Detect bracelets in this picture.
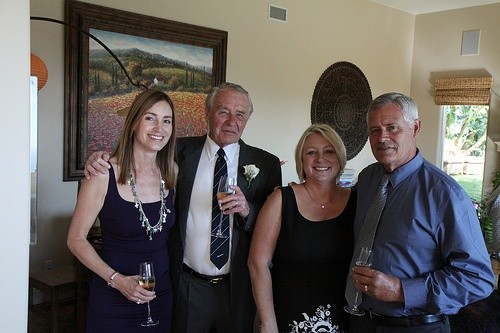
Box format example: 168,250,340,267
107,272,119,286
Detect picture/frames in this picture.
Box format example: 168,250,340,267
62,0,227,182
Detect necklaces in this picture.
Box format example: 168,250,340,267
127,174,171,241
321,206,325,209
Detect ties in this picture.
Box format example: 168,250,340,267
209,148,233,270
343,174,392,309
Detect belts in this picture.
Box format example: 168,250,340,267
364,315,444,329
183,264,232,286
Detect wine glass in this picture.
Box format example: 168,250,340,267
210,177,237,239
139,262,159,327
343,247,372,316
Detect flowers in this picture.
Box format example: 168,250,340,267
243,164,259,190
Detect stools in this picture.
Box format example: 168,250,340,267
27,267,72,333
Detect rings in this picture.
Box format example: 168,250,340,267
235,201,238,207
365,285,367,292
136,299,140,305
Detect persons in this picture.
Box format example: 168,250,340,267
83,66,282,333
67,91,180,333
247,123,357,333
288,92,495,333
